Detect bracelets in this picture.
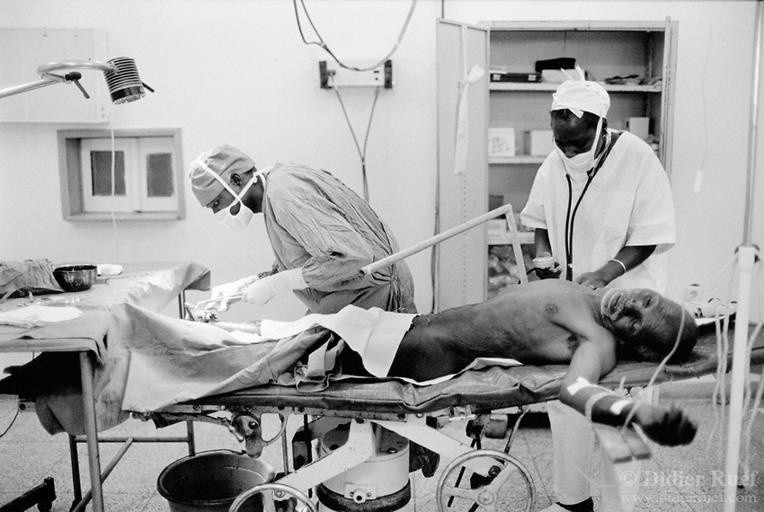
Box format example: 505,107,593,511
609,256,625,272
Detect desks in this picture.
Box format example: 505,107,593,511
0,265,211,511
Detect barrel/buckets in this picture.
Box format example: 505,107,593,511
155,448,275,512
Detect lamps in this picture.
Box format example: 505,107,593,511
0,57,154,106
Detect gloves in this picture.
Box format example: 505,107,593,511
203,272,262,315
240,267,308,308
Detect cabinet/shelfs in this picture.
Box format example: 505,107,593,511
434,18,677,319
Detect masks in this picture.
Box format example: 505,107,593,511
551,115,608,172
201,160,259,233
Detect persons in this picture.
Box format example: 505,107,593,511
328,280,700,448
189,144,415,316
517,81,677,510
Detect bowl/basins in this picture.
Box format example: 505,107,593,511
52,266,97,291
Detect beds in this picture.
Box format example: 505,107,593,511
134,317,755,512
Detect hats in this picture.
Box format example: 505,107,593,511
548,79,612,121
190,145,256,209
